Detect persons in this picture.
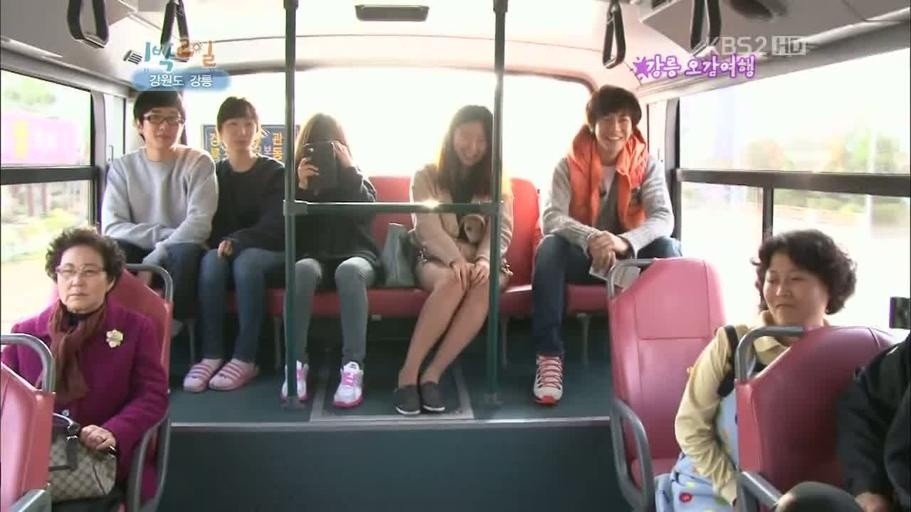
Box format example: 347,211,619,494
182,96,286,391
839,331,910,509
282,112,383,408
673,231,856,510
391,105,514,416
0,225,170,511
531,82,678,404
100,89,220,383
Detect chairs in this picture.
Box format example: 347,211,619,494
735,325,898,512
607,256,727,512
1,333,57,512
54,263,175,512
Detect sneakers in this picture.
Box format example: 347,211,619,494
420,379,446,413
180,358,223,394
531,354,565,406
391,375,419,416
332,367,365,409
208,356,261,391
281,360,307,403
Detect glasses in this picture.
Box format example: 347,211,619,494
141,112,184,127
52,264,106,277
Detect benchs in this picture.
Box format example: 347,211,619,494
152,177,623,368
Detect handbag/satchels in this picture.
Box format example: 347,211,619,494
45,410,117,501
380,221,413,291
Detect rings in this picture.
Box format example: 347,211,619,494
97,434,106,441
